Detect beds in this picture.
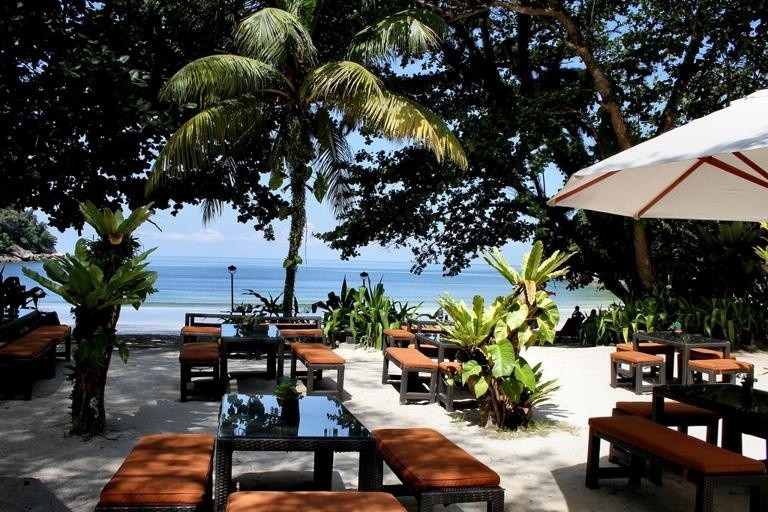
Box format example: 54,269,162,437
546,87,767,229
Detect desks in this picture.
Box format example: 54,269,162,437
359,271,369,288
227,264,238,311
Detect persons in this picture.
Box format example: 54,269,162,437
571,306,585,322
583,309,597,326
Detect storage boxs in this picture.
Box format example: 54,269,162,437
96,431,217,512
175,313,347,402
584,320,767,512
225,488,408,511
369,426,505,512
383,317,493,411
0,310,72,398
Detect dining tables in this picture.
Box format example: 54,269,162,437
272,376,304,414
